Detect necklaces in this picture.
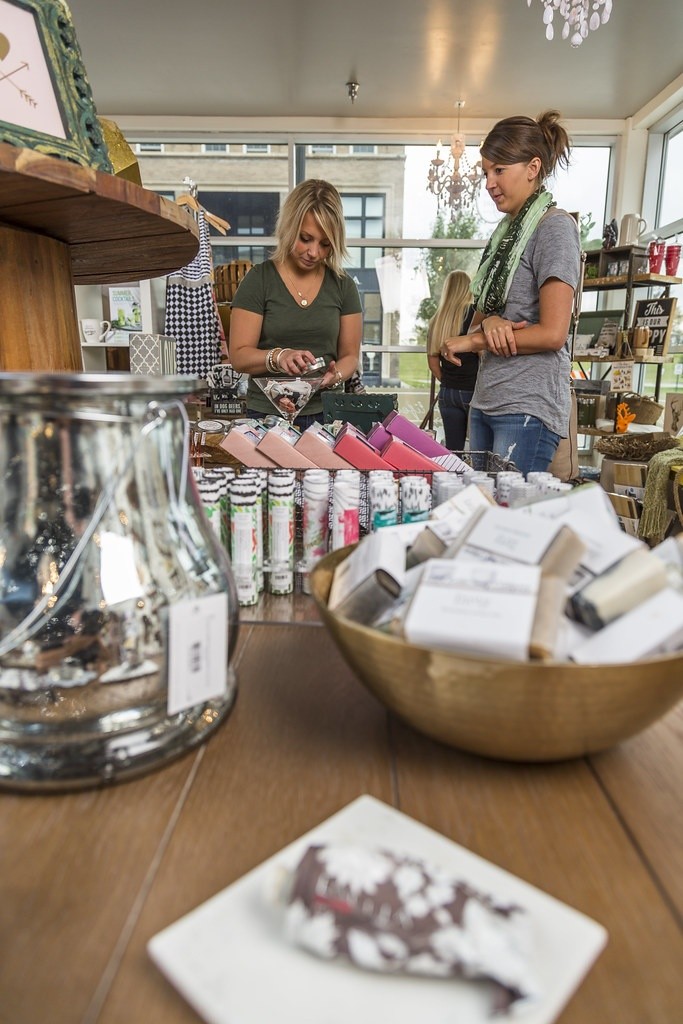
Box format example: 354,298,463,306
283,263,320,307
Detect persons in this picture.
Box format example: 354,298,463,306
438,110,581,488
426,270,478,458
229,179,363,432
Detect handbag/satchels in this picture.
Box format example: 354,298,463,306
607,392,664,425
545,388,580,483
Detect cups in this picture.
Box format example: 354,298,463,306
633,326,651,347
665,246,681,275
649,242,665,274
80,319,112,342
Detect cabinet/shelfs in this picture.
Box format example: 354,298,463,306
569,246,683,437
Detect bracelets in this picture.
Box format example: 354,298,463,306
266,348,291,374
480,322,484,333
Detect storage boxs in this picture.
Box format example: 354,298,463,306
219,409,477,468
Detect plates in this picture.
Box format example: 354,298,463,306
144,795,610,1024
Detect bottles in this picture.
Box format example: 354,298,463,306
0,370,239,792
619,214,647,245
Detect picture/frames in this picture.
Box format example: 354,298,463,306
0,1,113,175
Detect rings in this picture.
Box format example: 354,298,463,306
444,353,448,356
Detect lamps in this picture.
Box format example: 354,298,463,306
426,100,484,210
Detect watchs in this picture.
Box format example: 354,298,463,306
327,372,342,390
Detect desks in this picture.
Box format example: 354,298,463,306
0,593,682,1024
0,142,200,372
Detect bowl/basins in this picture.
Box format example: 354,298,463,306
307,543,682,763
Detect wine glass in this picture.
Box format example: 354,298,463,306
253,377,324,427
